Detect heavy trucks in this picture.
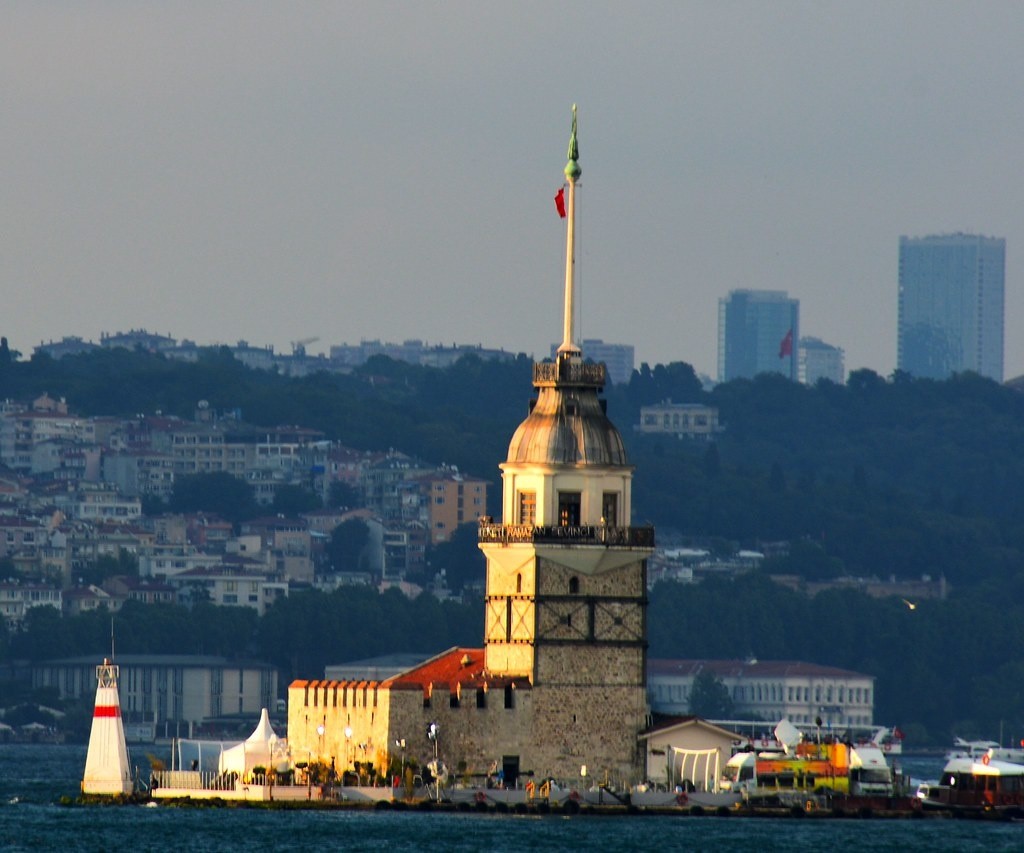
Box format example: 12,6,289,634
713,741,850,810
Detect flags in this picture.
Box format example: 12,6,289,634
554,188,568,219
779,330,792,358
895,729,907,741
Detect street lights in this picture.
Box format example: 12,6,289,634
429,721,440,803
344,726,354,771
317,725,326,762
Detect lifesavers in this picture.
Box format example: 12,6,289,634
675,791,689,805
569,791,580,801
911,797,922,809
476,791,487,801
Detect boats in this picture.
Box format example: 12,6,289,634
946,739,1024,763
914,756,1024,822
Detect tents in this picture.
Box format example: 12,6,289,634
218,709,287,774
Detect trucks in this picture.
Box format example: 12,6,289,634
848,745,894,799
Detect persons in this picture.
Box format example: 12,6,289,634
709,774,715,793
720,775,732,793
